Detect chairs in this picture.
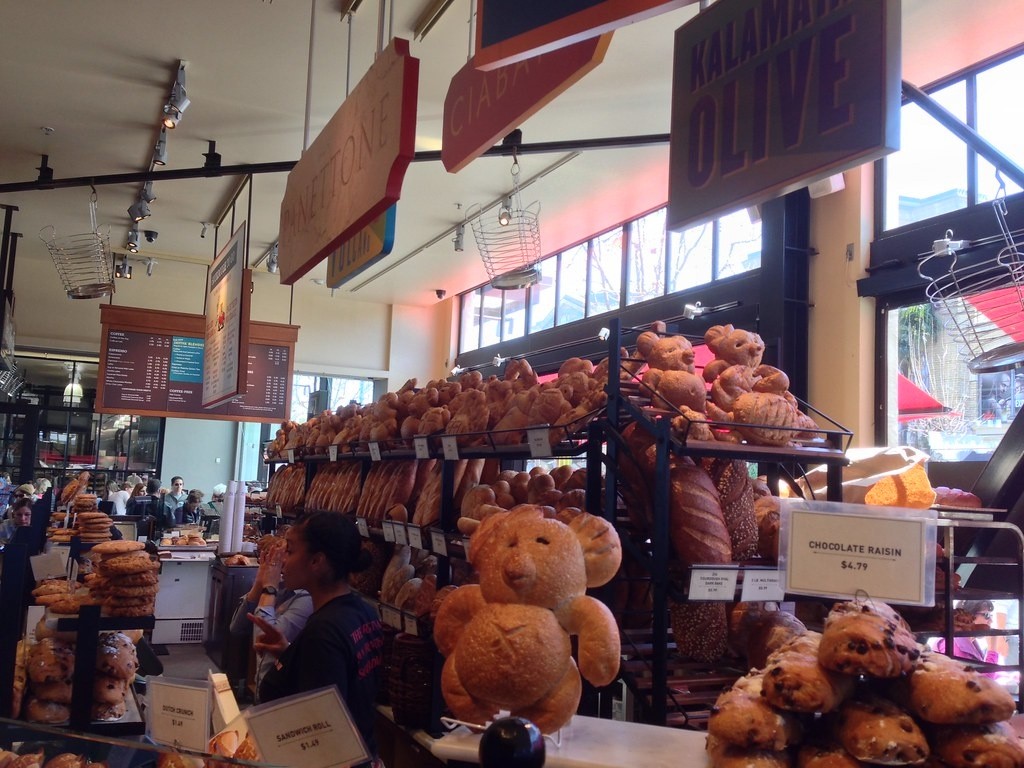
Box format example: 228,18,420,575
98,500,115,515
200,515,220,533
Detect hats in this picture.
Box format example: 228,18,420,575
186,493,201,503
213,483,227,496
142,475,148,480
147,479,162,491
14,484,34,496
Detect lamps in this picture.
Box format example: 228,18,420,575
497,194,514,226
266,241,280,274
452,226,465,252
63,368,83,408
114,83,190,278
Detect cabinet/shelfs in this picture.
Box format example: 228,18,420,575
254,319,854,728
4,605,164,744
930,504,1024,715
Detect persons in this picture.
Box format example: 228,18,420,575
1014,372,1024,400
981,373,1011,423
101,472,208,526
0,471,53,549
932,598,1002,682
209,481,227,505
228,510,390,768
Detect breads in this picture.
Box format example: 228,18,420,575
160,534,207,547
0,610,210,768
218,323,819,734
703,487,1024,768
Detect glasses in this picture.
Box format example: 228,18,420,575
14,494,26,498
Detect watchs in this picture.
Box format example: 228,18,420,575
259,585,277,597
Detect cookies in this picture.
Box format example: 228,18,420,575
30,480,159,619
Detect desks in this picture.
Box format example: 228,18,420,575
431,714,709,768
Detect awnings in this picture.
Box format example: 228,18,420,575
898,370,955,424
